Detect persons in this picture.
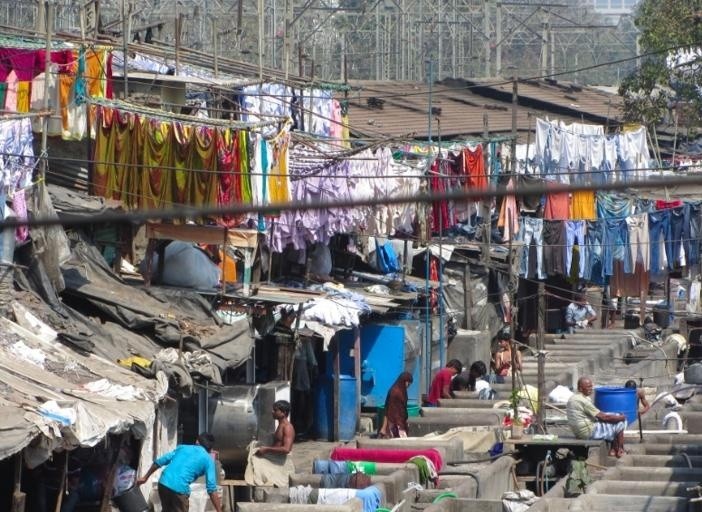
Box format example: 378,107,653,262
496,332,523,377
256,401,295,457
135,432,221,512
427,358,464,408
666,328,688,372
452,361,487,391
643,316,661,341
566,376,624,456
624,381,649,417
380,372,413,438
476,375,490,399
566,292,597,330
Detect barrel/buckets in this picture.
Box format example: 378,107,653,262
376,404,422,434
594,386,638,426
314,372,357,442
